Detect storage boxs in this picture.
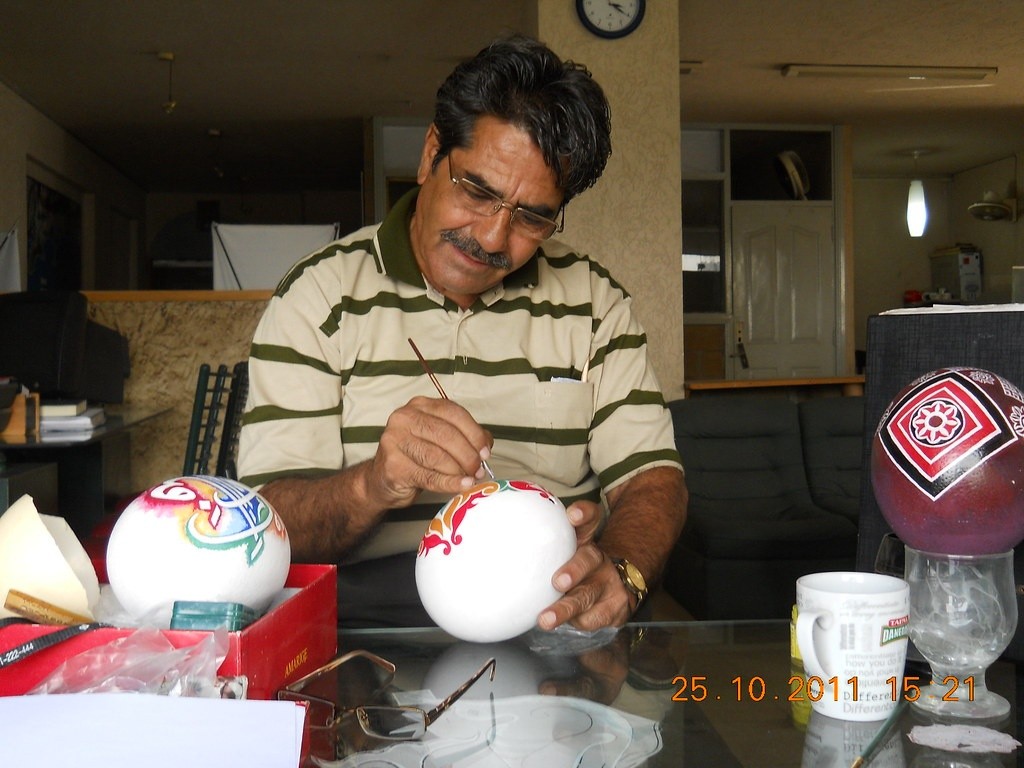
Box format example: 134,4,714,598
0,555,339,768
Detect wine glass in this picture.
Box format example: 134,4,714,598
903,544,1020,719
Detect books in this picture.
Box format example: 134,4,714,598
38,400,106,443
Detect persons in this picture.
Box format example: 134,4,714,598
236,35,695,635
540,628,684,768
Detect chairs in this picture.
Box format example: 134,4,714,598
659,387,859,621
183,360,249,487
799,391,866,519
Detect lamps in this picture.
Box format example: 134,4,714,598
782,64,998,83
894,146,940,238
158,51,177,114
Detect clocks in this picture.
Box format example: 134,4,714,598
576,0,646,40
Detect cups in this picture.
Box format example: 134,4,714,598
795,570,911,721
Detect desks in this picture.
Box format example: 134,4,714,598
0,402,177,540
310,624,1018,768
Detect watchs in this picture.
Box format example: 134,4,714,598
630,627,647,658
610,557,649,614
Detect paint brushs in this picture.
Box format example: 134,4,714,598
408,337,498,480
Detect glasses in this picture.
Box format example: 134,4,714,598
276,650,498,741
447,150,565,243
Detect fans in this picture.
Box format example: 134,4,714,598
967,186,1018,224
